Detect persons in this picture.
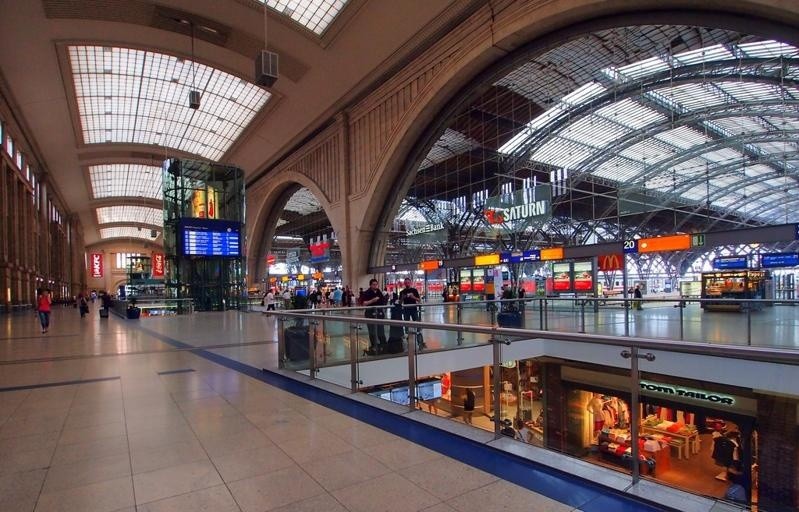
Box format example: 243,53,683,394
514,420,535,443
256,278,427,349
602,397,627,427
427,401,438,414
634,285,644,310
37,288,114,334
441,373,449,394
193,191,200,215
442,278,526,311
461,384,477,424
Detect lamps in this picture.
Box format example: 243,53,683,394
188,24,201,109
253,0,281,90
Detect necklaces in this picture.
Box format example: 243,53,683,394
501,419,516,437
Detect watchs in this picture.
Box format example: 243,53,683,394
586,394,604,434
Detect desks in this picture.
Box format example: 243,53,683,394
641,421,700,460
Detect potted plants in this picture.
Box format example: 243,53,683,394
497,288,521,328
126,297,141,319
283,292,316,361
